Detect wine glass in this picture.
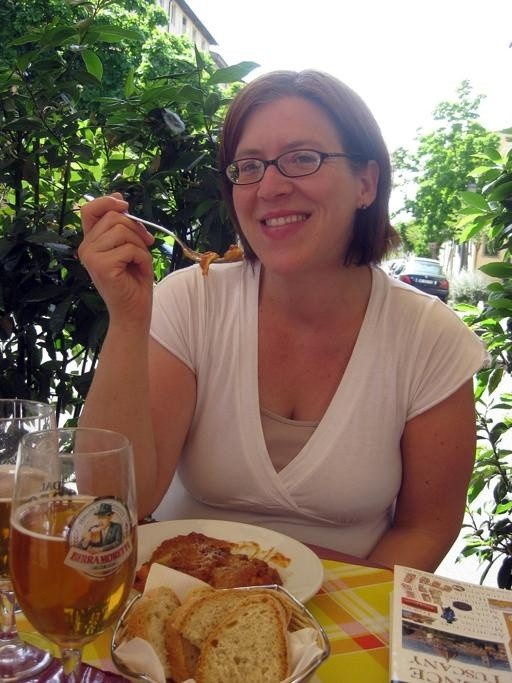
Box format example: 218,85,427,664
9,427,137,683
0,397,61,683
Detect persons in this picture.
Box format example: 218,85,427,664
74,70,488,579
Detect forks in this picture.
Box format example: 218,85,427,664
84,195,244,265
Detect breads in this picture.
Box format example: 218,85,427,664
115,583,292,683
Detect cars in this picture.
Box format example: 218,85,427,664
374,252,449,307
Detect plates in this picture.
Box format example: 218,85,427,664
132,519,323,606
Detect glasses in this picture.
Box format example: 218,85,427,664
225,148,367,185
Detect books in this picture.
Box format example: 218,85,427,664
386,560,512,683
